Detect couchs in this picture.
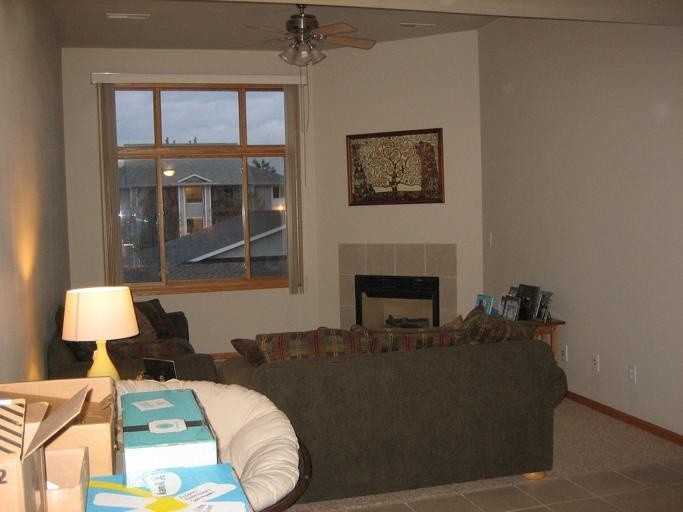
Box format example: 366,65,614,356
215,339,573,505
46,312,218,382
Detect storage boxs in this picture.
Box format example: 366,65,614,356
0,377,256,512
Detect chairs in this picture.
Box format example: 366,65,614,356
116,378,313,512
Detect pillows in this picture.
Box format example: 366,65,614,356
55,298,196,365
230,303,527,364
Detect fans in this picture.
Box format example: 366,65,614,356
233,3,377,50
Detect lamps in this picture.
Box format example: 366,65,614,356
62,286,141,379
278,40,326,66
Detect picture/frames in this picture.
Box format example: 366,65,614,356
345,127,445,206
476,284,554,322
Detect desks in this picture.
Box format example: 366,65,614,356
516,317,565,366
